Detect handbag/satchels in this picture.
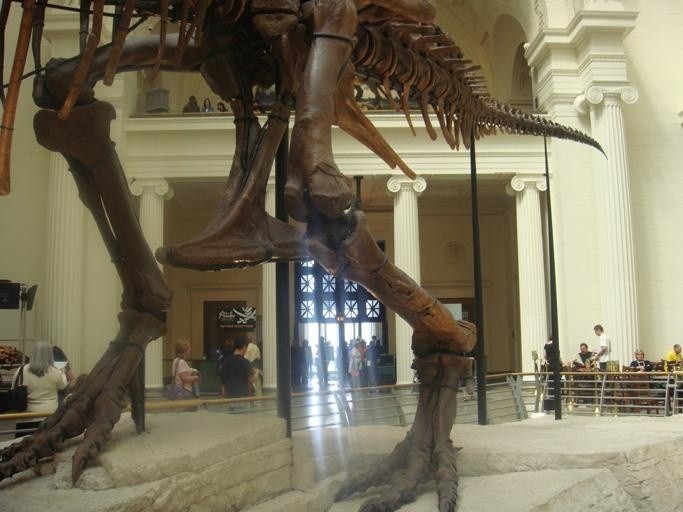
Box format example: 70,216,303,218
1,365,27,413
166,385,198,412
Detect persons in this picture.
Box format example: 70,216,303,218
290,335,383,387
666,343,683,365
216,336,263,398
12,341,68,439
591,325,610,370
182,95,228,113
572,343,596,368
172,340,200,399
52,345,75,403
630,349,652,371
543,335,563,395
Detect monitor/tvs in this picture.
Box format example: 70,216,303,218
0,283,20,309
26,285,37,310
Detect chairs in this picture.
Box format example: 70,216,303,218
539,358,683,415
